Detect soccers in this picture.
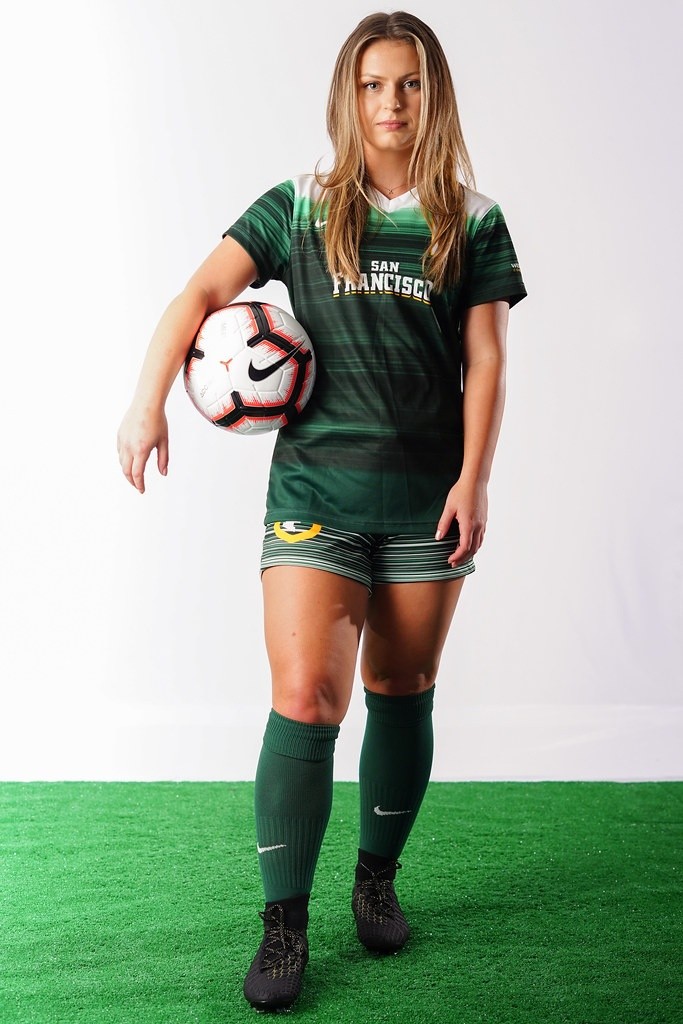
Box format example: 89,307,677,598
183,301,315,436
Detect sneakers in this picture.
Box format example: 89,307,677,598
347,847,410,951
243,893,312,1010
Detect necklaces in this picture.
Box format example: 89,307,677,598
373,182,407,194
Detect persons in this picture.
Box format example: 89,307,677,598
116,11,527,1010
